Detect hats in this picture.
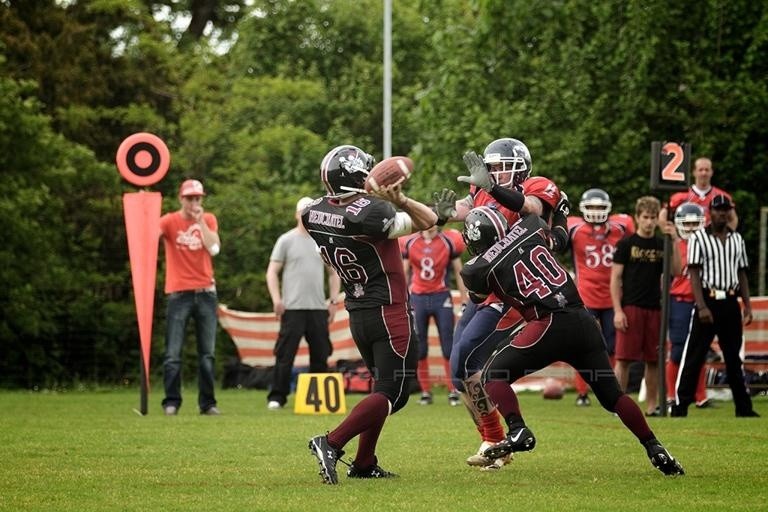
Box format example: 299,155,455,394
297,197,315,212
181,178,204,199
709,195,734,211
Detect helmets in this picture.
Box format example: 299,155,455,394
460,206,512,255
320,144,375,203
675,203,705,241
578,189,613,225
484,137,532,191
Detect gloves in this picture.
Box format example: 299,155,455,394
458,152,494,194
434,188,458,220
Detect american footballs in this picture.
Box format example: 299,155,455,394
365,156,414,195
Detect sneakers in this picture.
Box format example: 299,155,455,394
484,427,536,459
417,394,461,406
647,445,684,476
646,400,761,420
479,453,514,470
347,461,401,478
467,440,508,466
575,394,591,407
309,434,345,486
163,401,287,418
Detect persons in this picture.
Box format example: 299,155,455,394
266,138,684,488
658,157,761,419
159,179,221,416
